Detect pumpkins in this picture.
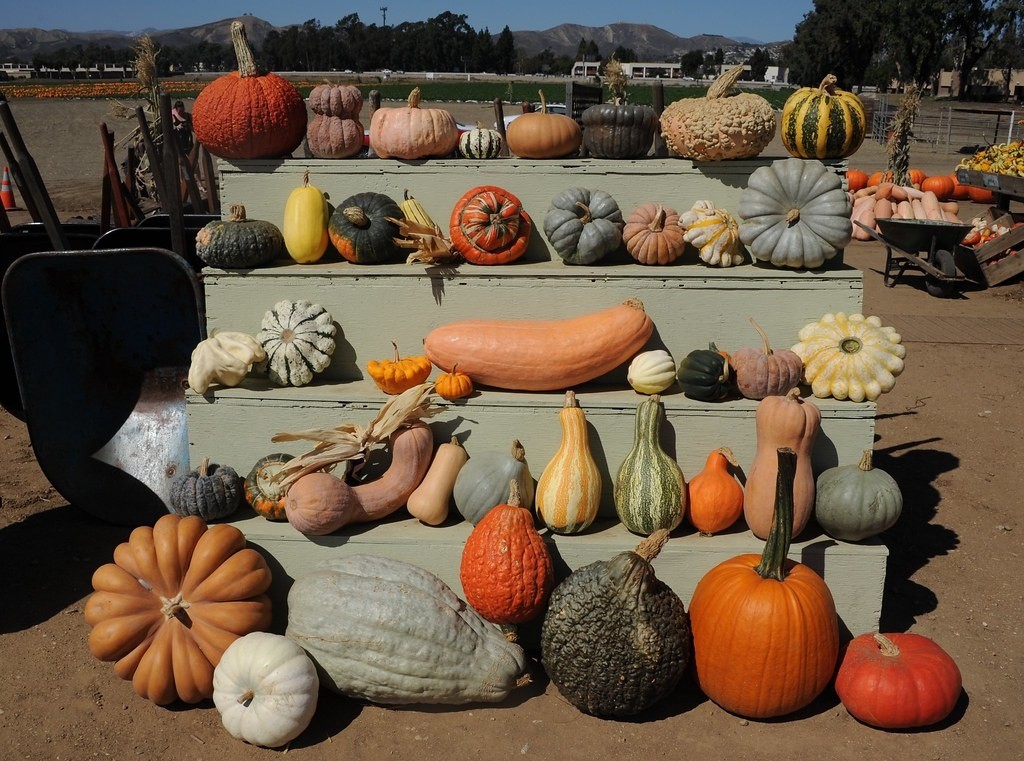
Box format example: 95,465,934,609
85,22,1023,744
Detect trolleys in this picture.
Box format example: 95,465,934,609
853,217,977,298
956,169,1024,213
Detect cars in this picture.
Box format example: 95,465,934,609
494,103,567,133
683,75,695,81
381,68,393,74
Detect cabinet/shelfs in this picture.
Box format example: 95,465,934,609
182,152,891,639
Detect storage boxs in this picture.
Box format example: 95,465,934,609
959,224,1024,287
964,205,1014,233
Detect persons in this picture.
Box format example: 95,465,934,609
171,100,209,193
